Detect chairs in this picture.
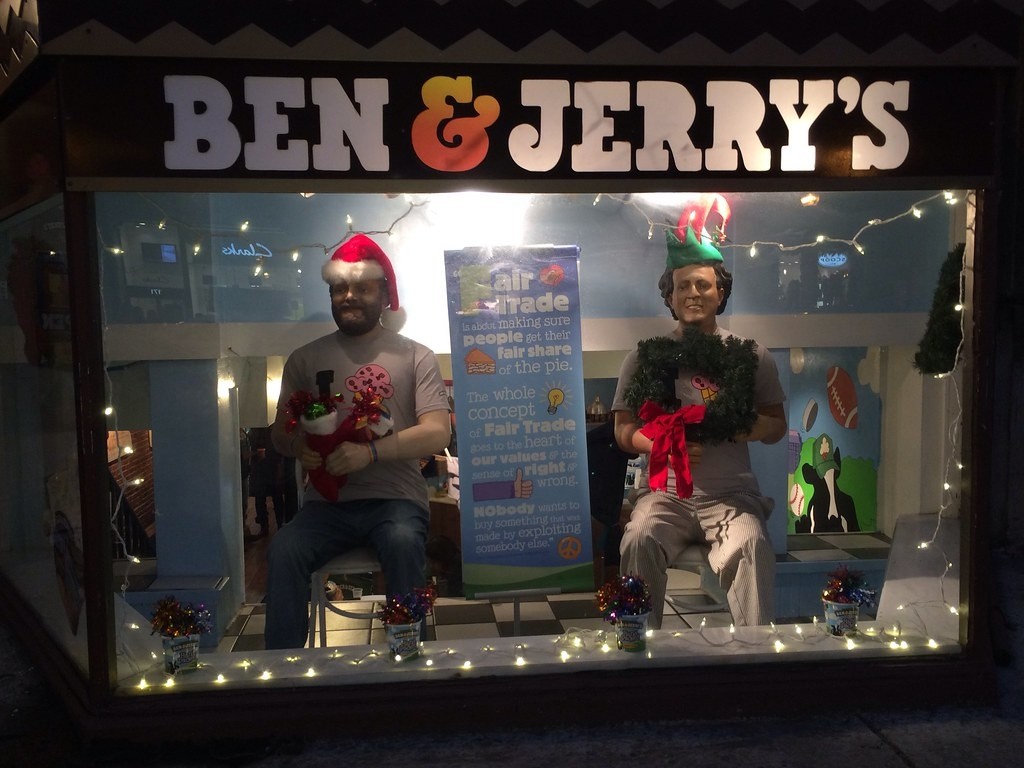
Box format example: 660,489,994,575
290,456,430,650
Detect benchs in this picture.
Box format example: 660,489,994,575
111,575,232,649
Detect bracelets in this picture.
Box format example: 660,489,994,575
366,441,381,464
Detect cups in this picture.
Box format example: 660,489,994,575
428,456,449,497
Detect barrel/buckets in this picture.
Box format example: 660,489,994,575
160,633,200,676
821,597,861,640
614,612,651,654
382,619,423,661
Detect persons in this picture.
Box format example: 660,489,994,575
611,217,789,627
262,235,453,651
248,428,284,538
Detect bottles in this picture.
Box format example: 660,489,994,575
255,447,266,463
